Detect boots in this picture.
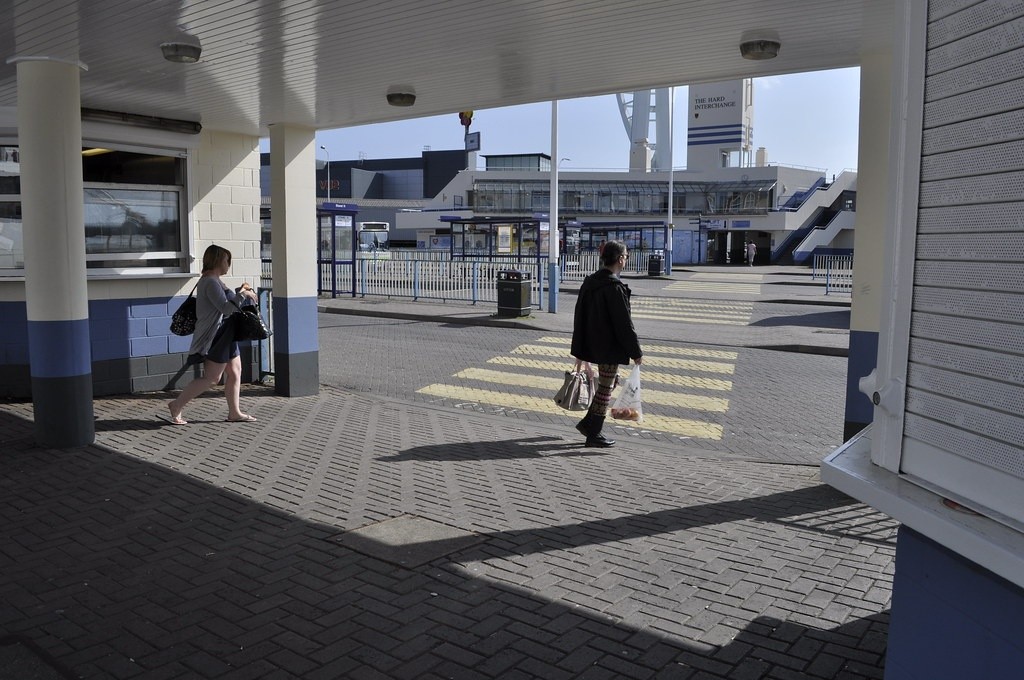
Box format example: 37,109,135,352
576,409,616,447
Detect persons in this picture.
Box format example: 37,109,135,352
570,239,644,448
748,240,756,267
156,245,259,424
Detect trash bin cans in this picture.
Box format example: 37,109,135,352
648,254,665,276
496,271,533,318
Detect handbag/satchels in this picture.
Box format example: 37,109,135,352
229,298,273,343
170,296,197,336
610,363,643,421
553,356,600,411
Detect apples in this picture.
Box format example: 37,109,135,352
611,407,639,420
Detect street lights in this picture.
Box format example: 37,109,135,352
320,145,331,203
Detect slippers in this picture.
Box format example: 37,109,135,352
153,414,187,426
224,414,257,422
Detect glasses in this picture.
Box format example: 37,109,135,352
622,253,629,259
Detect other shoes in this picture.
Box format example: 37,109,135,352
749,264,753,267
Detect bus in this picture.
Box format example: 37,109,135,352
262,222,391,263
